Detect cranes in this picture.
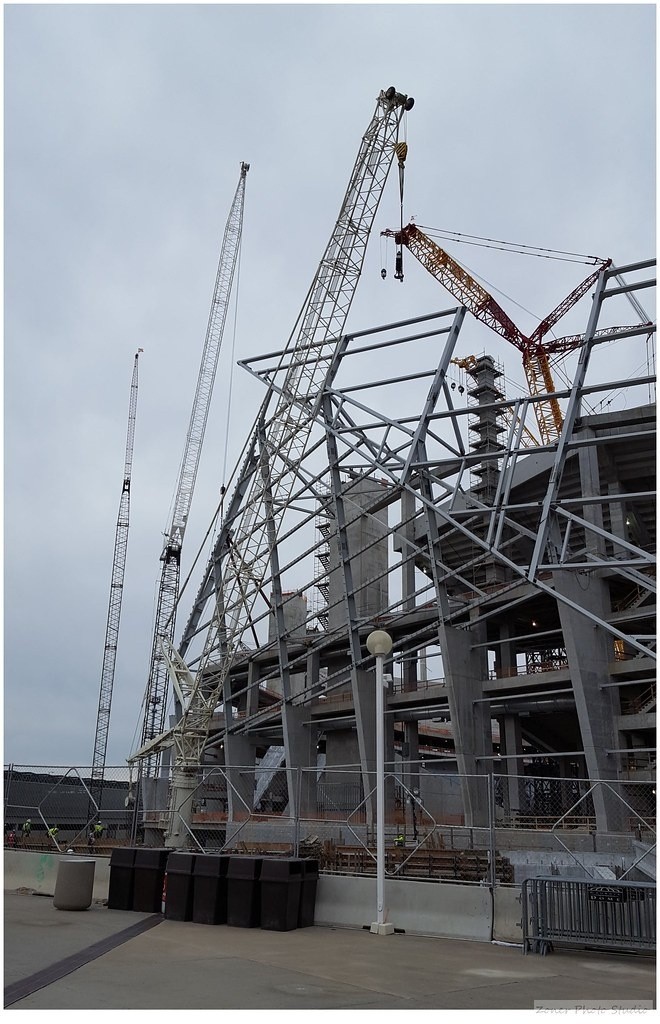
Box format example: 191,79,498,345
125,82,412,851
379,217,613,450
83,347,148,835
138,160,253,785
447,347,540,450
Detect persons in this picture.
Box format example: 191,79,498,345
45,827,60,839
94,820,104,839
22,818,32,839
8,829,18,849
87,832,96,854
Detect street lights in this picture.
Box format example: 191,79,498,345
358,629,396,936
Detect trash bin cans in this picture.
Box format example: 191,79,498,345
107,846,138,911
132,847,167,913
164,850,321,931
